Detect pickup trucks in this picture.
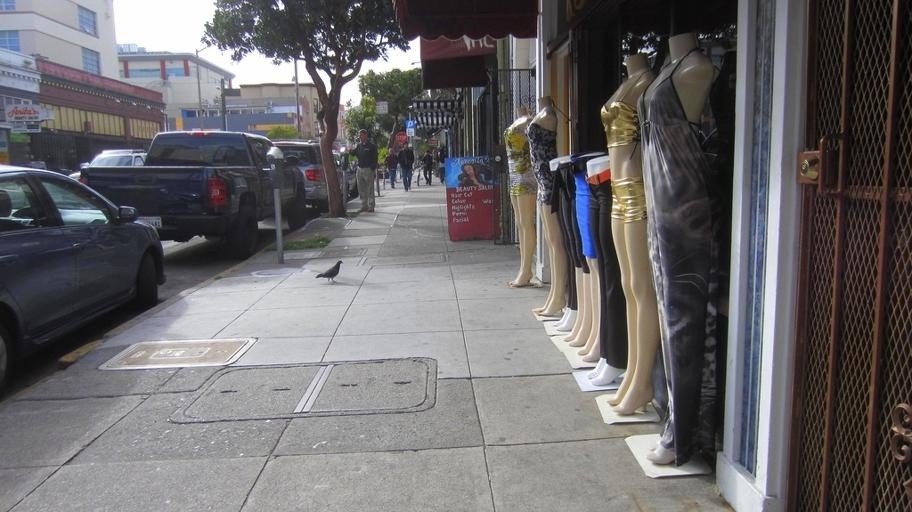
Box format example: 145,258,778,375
80,129,305,260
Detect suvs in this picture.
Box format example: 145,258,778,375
270,139,349,206
69,150,148,180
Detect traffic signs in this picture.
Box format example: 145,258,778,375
406,120,414,128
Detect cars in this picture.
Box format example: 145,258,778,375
0,164,164,395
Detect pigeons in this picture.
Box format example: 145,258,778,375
315,260,343,283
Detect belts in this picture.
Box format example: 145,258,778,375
585,169,611,186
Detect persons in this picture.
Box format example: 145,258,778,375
504,108,538,287
438,145,447,184
526,96,568,316
346,129,378,212
433,149,438,176
569,153,607,363
422,150,433,186
384,148,398,188
398,141,414,191
549,156,578,331
560,153,592,347
587,156,628,387
461,164,491,187
601,53,660,415
637,34,714,468
